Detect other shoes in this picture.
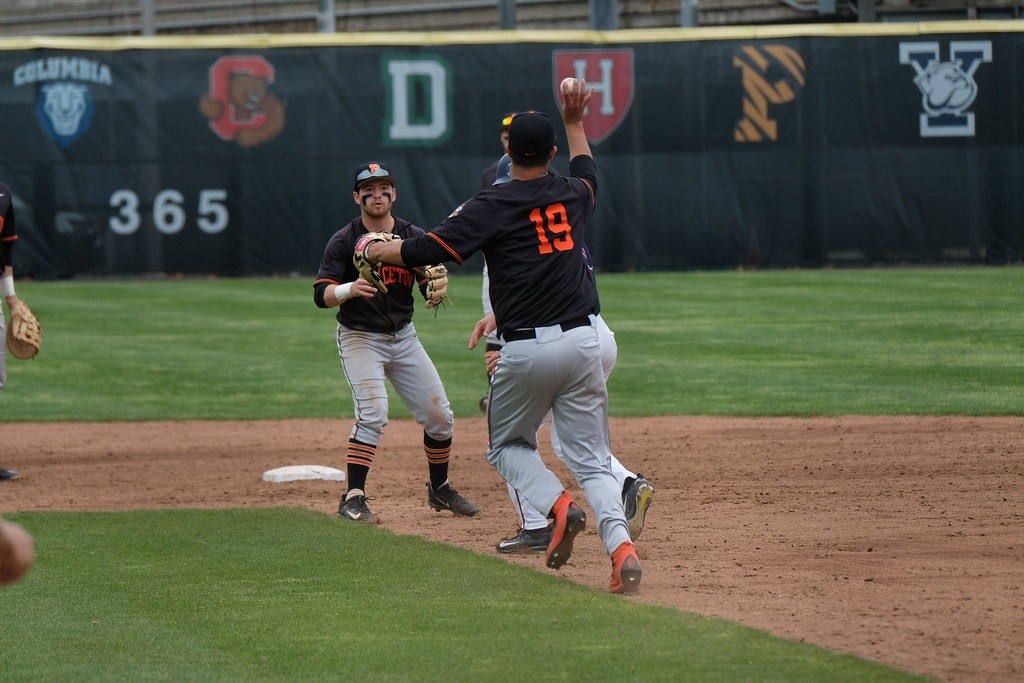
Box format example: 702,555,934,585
0,467,22,480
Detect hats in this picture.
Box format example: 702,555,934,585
507,110,557,158
500,112,519,133
353,162,394,192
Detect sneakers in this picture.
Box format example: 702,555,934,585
608,541,643,594
496,520,551,553
544,490,586,570
425,478,482,516
337,494,380,525
621,473,655,542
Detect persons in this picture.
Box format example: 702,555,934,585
0,181,43,480
0,516,34,587
353,77,657,594
312,161,480,522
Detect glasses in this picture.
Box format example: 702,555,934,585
501,113,518,127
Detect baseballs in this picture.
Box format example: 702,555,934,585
560,77,580,96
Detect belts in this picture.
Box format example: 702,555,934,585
503,319,582,343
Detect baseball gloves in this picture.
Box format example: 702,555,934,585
413,262,455,320
6,299,44,363
351,221,414,294
